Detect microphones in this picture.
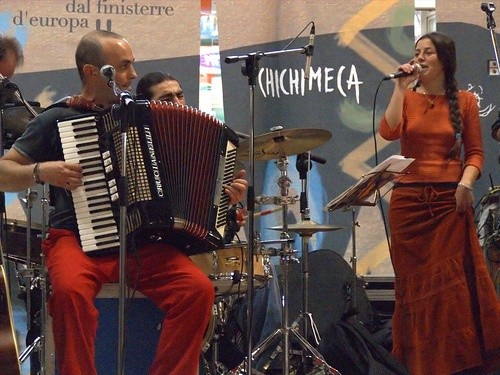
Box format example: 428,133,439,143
384,64,422,80
304,26,315,80
100,65,116,78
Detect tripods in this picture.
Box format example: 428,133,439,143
221,149,344,375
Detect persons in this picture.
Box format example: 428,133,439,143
378,32,500,375
136,71,283,375
0,30,248,375
0,36,23,80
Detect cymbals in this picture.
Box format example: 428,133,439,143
236,128,332,160
265,217,345,237
0,107,48,150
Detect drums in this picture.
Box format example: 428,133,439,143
0,218,48,265
188,239,273,296
0,264,21,375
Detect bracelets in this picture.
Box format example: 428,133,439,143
458,183,473,190
33,162,44,185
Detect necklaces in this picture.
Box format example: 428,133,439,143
421,88,444,108
